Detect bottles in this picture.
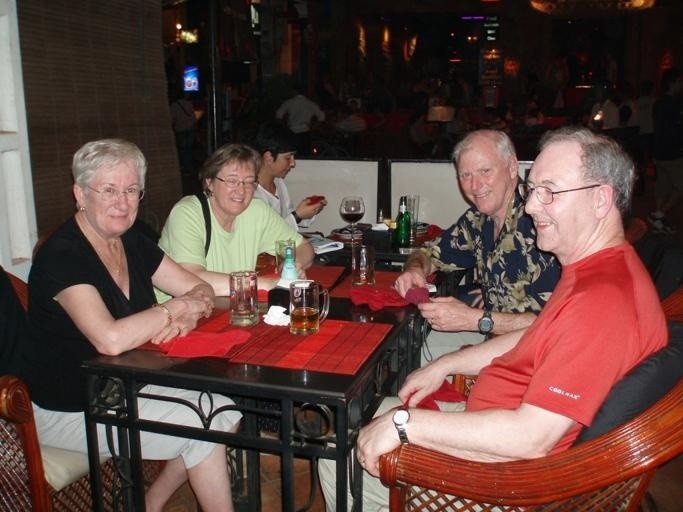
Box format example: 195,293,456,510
395,196,410,248
281,247,298,281
378,208,384,226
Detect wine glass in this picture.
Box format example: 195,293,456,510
338,195,364,247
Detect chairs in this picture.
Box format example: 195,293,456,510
0,267,166,512
379,283,682,512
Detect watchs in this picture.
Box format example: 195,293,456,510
392,406,415,444
479,310,493,336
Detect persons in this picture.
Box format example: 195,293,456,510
319,126,669,512
7,138,242,512
154,143,315,308
395,130,561,364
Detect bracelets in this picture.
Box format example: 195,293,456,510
151,302,172,324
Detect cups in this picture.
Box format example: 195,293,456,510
288,281,330,336
350,240,376,287
268,288,289,315
405,195,418,243
275,239,296,278
228,271,259,327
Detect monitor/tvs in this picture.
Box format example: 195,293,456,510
182,62,201,92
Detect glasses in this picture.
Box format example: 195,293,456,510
86,183,144,202
517,183,601,205
214,175,259,190
